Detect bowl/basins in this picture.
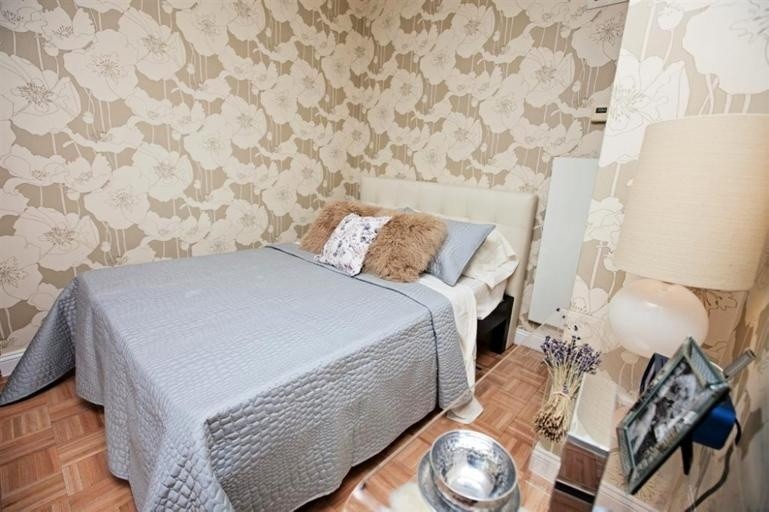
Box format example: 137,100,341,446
429,425,520,508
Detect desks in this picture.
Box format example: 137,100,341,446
340,304,652,512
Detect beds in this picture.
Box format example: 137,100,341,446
64,176,540,512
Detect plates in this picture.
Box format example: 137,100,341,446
409,441,525,512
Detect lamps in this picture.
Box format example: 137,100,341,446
606,112,769,409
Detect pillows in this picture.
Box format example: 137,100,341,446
301,199,520,292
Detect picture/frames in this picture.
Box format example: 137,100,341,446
609,335,732,497
